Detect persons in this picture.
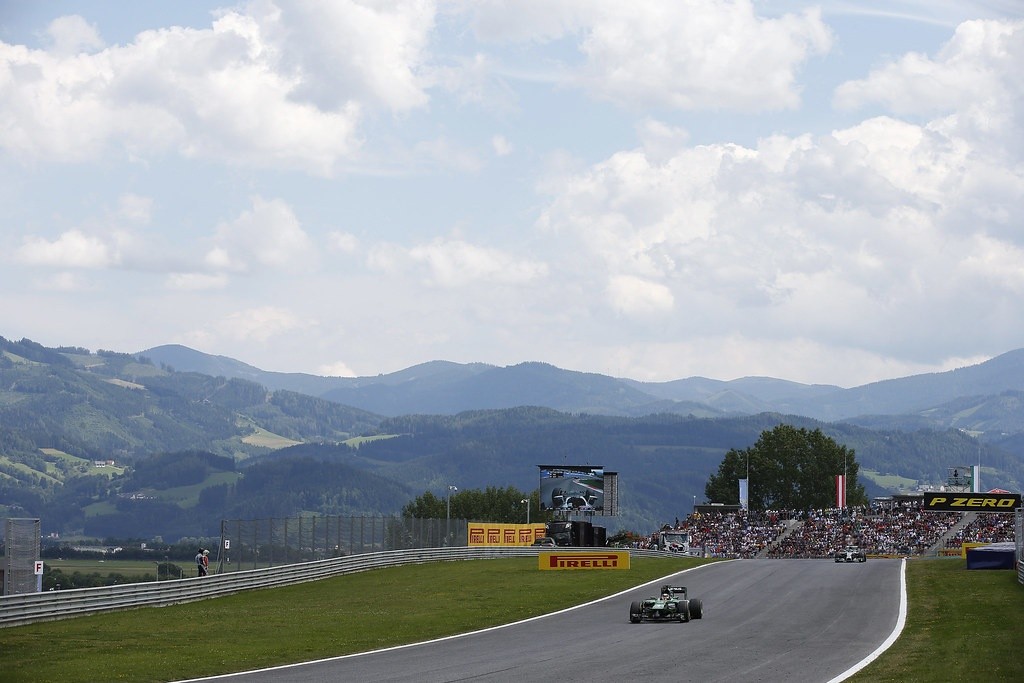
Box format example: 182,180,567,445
660,592,671,600
195,547,210,576
615,499,1024,559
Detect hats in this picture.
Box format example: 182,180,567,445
203,550,210,556
198,547,204,552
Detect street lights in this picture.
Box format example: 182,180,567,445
694,496,697,505
446,485,458,547
520,499,530,525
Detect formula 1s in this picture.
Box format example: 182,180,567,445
835,545,867,564
628,583,702,623
